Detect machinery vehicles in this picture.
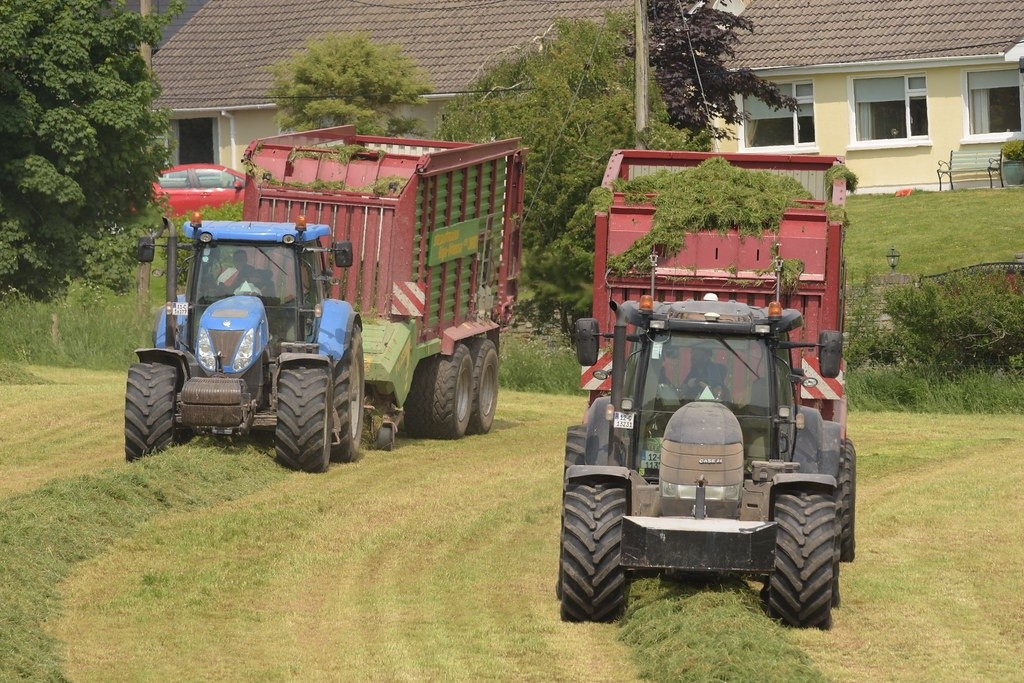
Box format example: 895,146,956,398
557,150,855,628
124,123,526,474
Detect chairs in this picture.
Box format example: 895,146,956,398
257,268,276,296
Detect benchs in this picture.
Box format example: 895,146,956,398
937,150,1004,192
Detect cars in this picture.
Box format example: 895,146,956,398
152,162,247,217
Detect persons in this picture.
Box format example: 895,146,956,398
220,249,256,293
680,348,726,389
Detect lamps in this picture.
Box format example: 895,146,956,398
886,246,901,274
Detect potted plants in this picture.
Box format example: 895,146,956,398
1001,138,1024,185
